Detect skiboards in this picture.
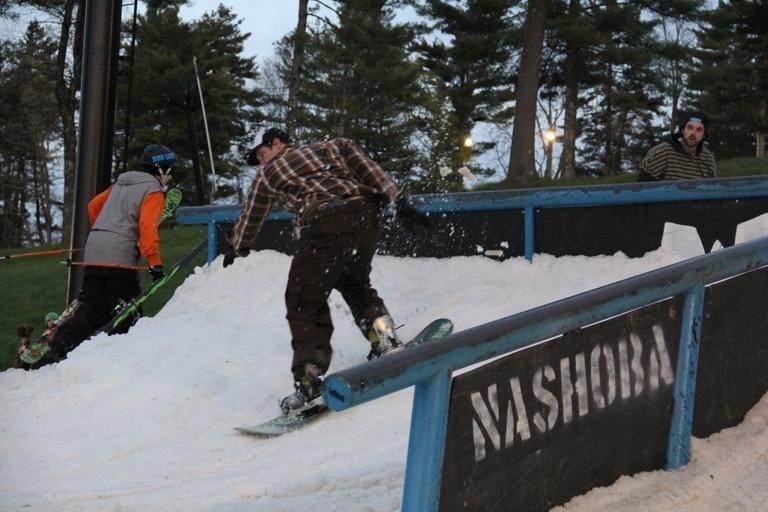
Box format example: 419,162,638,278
19,187,208,364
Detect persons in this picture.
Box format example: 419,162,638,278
223,128,432,412
637,111,717,181
70,144,179,344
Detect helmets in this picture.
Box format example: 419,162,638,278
142,144,176,176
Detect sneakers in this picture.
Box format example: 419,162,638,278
278,377,327,419
367,333,404,361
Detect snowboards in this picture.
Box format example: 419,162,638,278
234,318,454,436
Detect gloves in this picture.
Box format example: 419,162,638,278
222,246,237,267
150,265,163,280
395,197,433,233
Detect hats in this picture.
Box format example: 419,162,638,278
682,111,709,132
243,126,289,166
